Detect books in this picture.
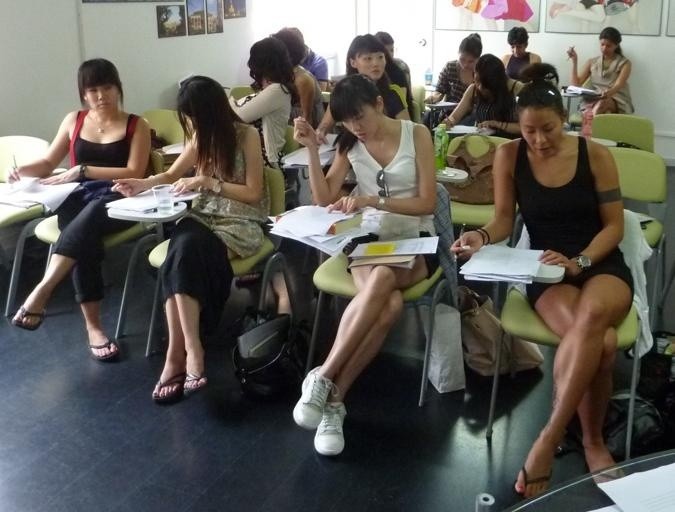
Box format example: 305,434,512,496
346,257,416,269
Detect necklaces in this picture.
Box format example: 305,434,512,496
90,111,121,133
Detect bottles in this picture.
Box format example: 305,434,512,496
582,105,593,138
434,123,449,170
424,68,433,85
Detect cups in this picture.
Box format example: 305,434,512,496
152,183,175,215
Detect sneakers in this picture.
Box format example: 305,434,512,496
293,366,347,456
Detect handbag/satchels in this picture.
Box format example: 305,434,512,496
606,391,669,461
444,142,496,205
458,286,544,376
420,303,466,394
233,321,305,398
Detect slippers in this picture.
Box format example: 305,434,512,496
12,305,46,329
512,465,552,499
152,369,209,403
87,340,117,359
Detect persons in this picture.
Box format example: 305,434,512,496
222,37,294,287
292,74,440,460
109,74,269,403
446,59,635,499
442,54,526,140
567,27,635,115
314,32,413,152
423,33,483,106
280,28,327,93
499,27,541,81
7,57,153,361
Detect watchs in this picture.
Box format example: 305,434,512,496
574,254,592,271
214,180,222,194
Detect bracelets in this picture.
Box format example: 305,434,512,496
377,195,387,211
80,162,87,182
477,228,491,246
474,230,485,246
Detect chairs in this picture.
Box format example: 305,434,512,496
0,87,668,468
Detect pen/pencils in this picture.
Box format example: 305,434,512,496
459,224,466,263
146,202,181,214
12,153,18,170
445,114,454,128
